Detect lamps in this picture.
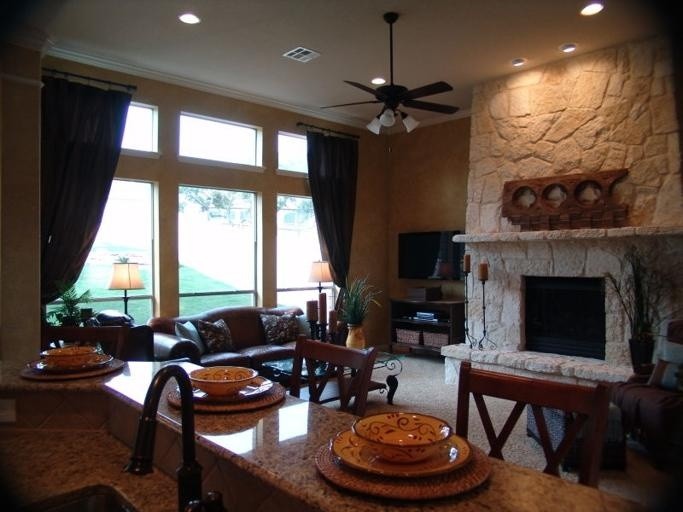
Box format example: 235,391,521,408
104,260,145,313
366,100,421,137
307,259,332,294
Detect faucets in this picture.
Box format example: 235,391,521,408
125,364,227,511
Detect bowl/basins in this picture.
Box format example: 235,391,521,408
188,366,259,393
353,411,449,462
38,345,99,369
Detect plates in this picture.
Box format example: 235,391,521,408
331,428,470,477
174,376,274,401
23,355,113,371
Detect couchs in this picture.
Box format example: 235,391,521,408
141,305,309,391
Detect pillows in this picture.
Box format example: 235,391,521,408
297,312,311,336
173,319,205,352
199,315,232,354
258,309,298,343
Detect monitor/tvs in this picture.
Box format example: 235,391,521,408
398,230,465,281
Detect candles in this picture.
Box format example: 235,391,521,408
315,291,326,327
465,253,470,274
477,259,489,282
329,308,336,334
306,297,318,324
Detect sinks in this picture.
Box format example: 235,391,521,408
23,483,141,511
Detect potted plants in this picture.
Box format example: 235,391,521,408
602,235,682,379
47,288,89,343
336,274,382,350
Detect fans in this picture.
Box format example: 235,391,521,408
316,10,461,118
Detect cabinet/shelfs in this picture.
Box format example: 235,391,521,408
389,292,466,358
126,324,154,361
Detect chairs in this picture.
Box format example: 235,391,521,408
448,360,611,487
286,330,375,416
612,307,682,478
39,326,128,363
